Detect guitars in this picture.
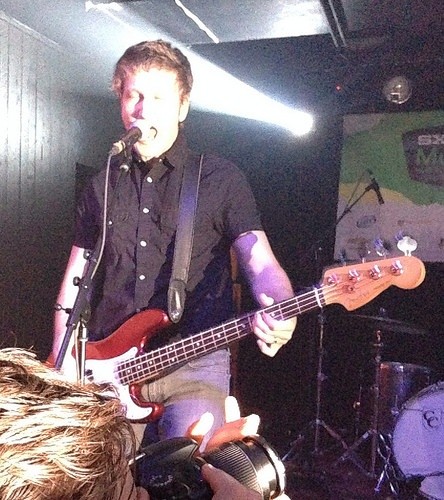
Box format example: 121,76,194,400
70,236,426,424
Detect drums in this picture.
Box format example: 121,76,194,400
373,359,441,436
379,381,444,499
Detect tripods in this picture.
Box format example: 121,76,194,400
278,308,394,500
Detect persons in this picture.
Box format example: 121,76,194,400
51,40,297,451
0,347,290,500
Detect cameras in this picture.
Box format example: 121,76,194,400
127,433,286,500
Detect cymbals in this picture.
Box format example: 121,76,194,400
336,305,426,334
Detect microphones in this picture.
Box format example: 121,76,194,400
112,119,150,155
369,169,384,205
